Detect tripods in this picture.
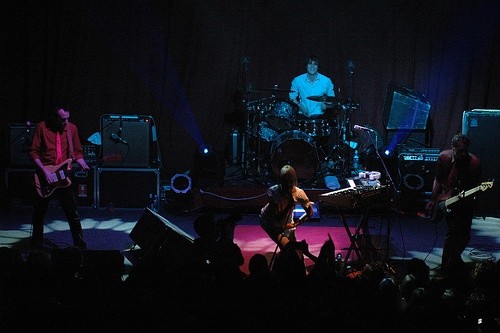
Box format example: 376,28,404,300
321,71,364,183
224,66,266,185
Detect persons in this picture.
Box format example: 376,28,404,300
261,165,313,248
289,56,335,119
427,134,481,283
0,214,500,333
32,106,89,248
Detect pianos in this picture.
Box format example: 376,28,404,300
318,183,393,271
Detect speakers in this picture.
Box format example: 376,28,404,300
401,155,439,193
130,207,195,256
460,110,500,213
8,126,52,168
102,119,153,166
95,166,161,210
69,250,125,286
4,167,59,206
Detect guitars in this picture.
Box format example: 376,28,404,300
35,154,126,198
426,179,493,225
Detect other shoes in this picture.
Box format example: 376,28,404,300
74,242,87,249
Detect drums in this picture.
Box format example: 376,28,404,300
256,119,279,142
301,118,333,136
270,130,320,183
267,96,298,129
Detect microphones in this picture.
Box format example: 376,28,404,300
287,187,293,202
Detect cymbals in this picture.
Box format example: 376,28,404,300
255,87,294,92
307,94,340,102
324,101,361,107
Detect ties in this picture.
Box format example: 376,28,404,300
55,130,62,166
